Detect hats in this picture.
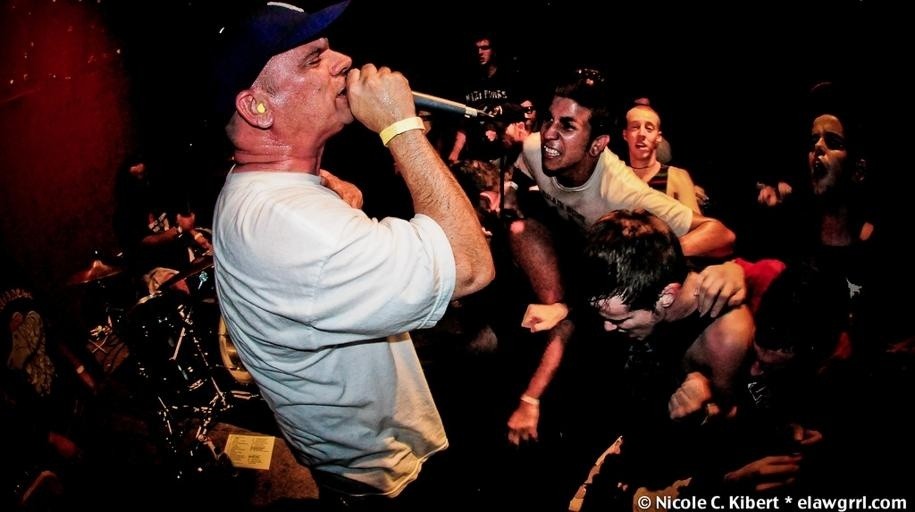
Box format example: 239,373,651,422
205,1,349,89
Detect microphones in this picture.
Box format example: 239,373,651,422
411,89,495,121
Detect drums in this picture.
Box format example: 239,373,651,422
181,292,259,392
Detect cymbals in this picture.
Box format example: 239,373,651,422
156,251,214,291
63,256,127,288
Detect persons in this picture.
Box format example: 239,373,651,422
3,1,915,512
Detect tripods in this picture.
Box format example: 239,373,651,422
102,282,267,486
